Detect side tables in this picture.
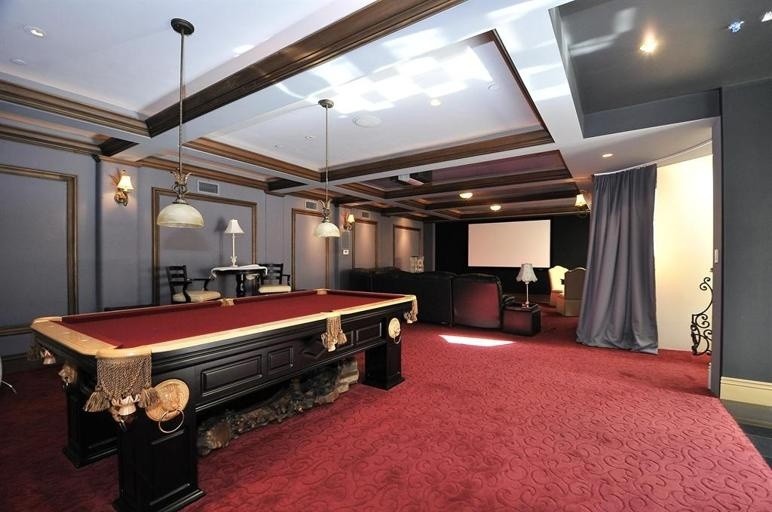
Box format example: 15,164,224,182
501,301,543,337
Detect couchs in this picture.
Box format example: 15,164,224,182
408,267,459,327
372,268,416,294
451,269,516,331
340,266,374,293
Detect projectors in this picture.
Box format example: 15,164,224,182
390,171,432,186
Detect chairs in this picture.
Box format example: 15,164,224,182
556,266,589,318
543,263,569,306
257,262,295,293
164,264,223,304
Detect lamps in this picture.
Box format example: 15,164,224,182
155,16,207,231
314,95,341,240
109,167,135,207
514,261,538,304
222,216,246,269
574,193,592,213
343,212,356,231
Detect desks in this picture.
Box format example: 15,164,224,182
209,262,269,298
27,286,424,511
